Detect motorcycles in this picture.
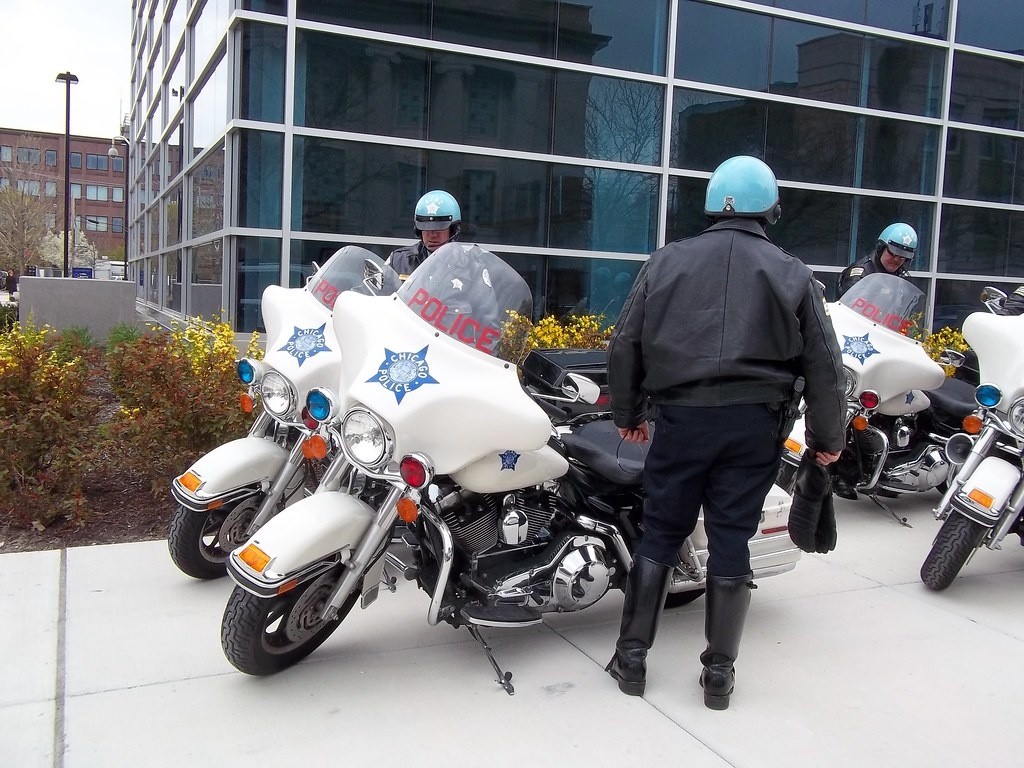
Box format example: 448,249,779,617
168,236,1024,696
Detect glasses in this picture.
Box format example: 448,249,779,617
887,247,906,260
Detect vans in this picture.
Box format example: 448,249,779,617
107,276,124,280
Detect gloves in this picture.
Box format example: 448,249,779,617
788,449,830,552
815,486,837,553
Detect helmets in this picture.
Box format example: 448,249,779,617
877,223,918,274
705,155,781,225
414,190,461,238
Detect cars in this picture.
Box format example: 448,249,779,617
0,271,9,290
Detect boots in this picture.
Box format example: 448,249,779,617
605,554,674,695
699,571,758,710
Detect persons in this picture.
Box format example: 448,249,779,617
833,223,918,499
559,266,634,326
5,269,18,297
604,152,850,711
380,189,503,353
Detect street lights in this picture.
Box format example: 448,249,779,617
55,71,80,280
